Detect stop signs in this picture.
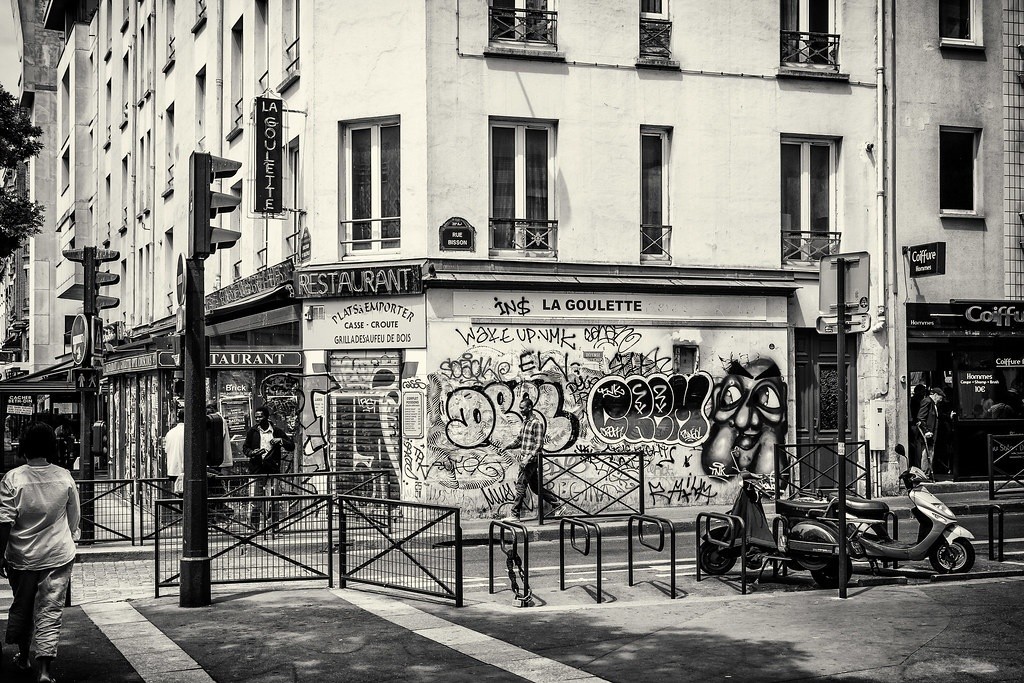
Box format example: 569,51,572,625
176,255,185,305
72,315,88,362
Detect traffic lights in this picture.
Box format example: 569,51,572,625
75,370,100,391
62,245,86,266
206,414,225,466
188,150,242,260
92,246,120,317
93,420,108,456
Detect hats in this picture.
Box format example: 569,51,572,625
177,410,185,420
931,388,946,397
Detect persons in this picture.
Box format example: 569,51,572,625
0,422,80,683
916,388,956,478
497,399,567,522
206,399,235,521
164,409,185,477
243,407,296,533
981,388,993,411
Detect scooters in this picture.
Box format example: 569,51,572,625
699,446,885,589
807,444,976,574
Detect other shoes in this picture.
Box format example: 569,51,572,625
248,527,258,533
41,675,55,683
265,530,283,539
555,506,566,517
501,515,520,522
13,653,30,669
